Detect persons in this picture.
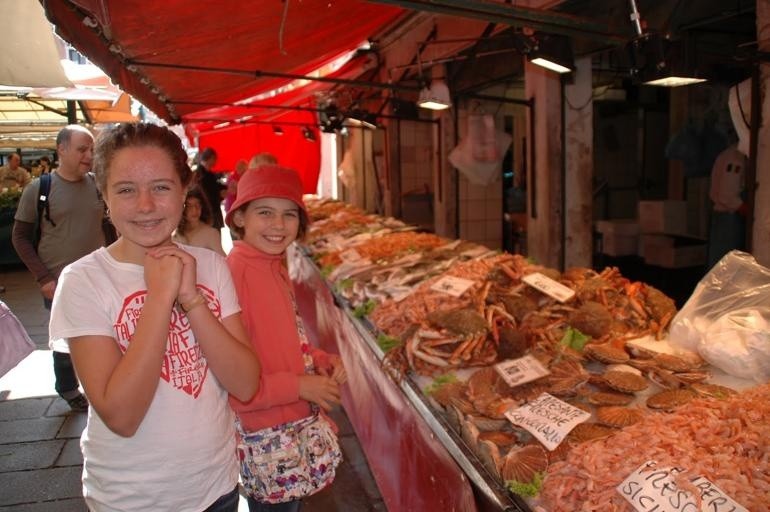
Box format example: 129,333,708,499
11,124,105,412
39,157,53,175
1,153,32,190
170,147,279,257
224,168,349,511
709,142,749,263
48,122,259,512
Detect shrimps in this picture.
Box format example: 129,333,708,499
369,251,513,338
534,384,770,512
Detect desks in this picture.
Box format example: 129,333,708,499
0,206,40,271
287,242,531,512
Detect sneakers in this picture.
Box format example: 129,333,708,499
55,381,89,411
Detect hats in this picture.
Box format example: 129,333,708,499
225,164,311,226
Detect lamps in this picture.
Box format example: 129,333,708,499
629,2,655,51
313,100,378,135
527,47,572,74
417,64,452,112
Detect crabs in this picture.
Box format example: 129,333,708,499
381,257,678,387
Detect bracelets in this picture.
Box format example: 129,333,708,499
182,295,206,310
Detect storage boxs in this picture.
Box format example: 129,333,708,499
597,199,708,268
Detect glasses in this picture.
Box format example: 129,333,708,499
186,201,201,210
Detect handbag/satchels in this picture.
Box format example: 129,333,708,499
237,413,342,506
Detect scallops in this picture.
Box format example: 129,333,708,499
431,336,739,484
